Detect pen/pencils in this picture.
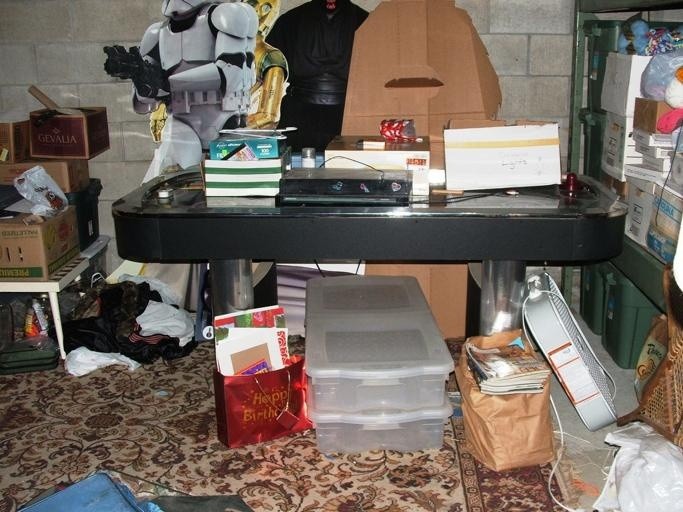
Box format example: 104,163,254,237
432,189,464,196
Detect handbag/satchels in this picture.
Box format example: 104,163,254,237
1,338,60,375
213,354,316,449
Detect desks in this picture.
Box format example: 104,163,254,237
110,170,627,343
0,257,90,359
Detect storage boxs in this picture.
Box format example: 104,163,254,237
308,380,453,455
29,106,110,159
632,96,670,134
442,115,563,189
601,111,642,182
646,185,683,264
204,146,292,196
324,134,430,195
579,256,614,336
600,51,653,118
624,183,654,250
0,120,30,163
599,260,661,369
64,178,102,251
0,205,80,281
304,275,454,411
0,160,89,193
81,236,109,282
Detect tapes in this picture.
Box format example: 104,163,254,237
156,187,174,199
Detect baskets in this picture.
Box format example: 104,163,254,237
617,263,683,448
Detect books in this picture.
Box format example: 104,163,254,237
210,305,291,374
465,338,549,395
